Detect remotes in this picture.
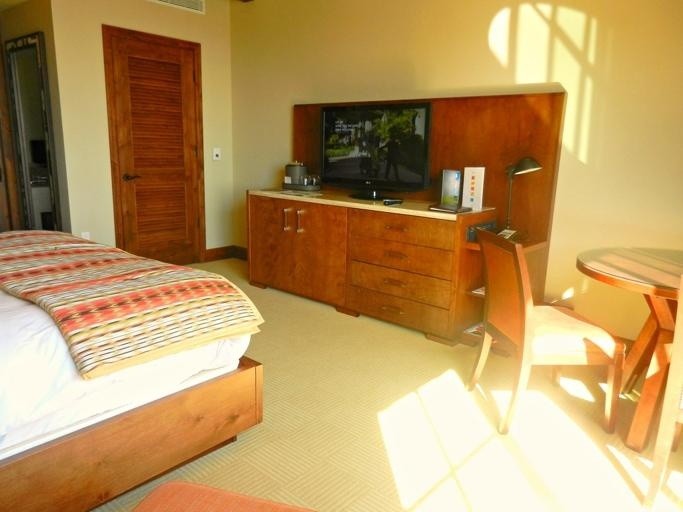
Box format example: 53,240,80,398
384,199,404,206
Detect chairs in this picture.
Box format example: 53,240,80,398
466,232,626,435
643,275,683,505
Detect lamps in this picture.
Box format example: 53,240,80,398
504,156,543,229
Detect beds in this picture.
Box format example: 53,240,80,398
0,230,265,512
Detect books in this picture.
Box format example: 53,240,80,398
428,204,471,214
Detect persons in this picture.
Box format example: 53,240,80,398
378,137,403,183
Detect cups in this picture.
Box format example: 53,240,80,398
300,175,321,186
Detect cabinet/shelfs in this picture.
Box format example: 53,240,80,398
347,204,496,347
246,189,346,314
454,239,548,357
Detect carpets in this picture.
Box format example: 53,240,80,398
131,481,317,512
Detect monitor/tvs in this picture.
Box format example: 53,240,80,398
320,101,431,200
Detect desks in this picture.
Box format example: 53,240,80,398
576,247,683,453
